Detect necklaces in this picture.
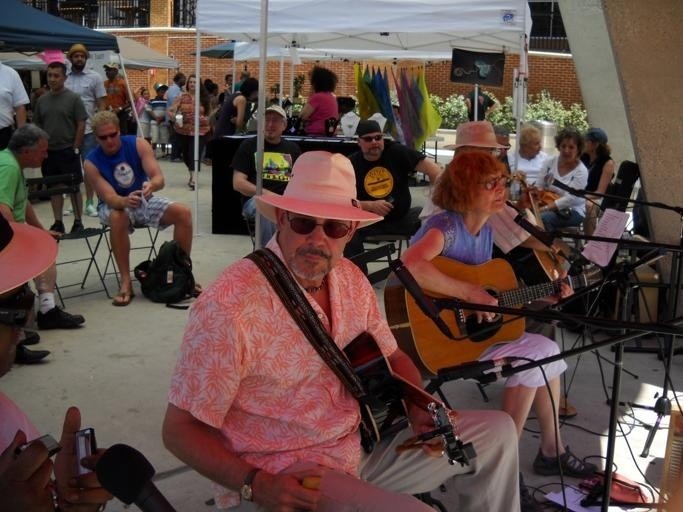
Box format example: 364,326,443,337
306,275,327,294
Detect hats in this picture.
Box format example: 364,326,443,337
67,44,91,61
582,128,608,144
103,61,119,71
266,104,289,118
253,151,384,225
443,121,509,148
359,120,381,137
0,212,58,294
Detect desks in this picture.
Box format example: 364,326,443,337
212,137,400,233
415,136,444,184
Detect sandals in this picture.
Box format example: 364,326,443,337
534,446,597,478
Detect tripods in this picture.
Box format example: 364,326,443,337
607,250,683,456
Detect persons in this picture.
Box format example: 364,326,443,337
492,125,614,241
82,112,204,306
1,214,115,511
233,106,302,247
1,44,131,234
341,121,442,275
336,97,360,135
133,71,259,188
416,120,578,419
297,66,339,134
163,151,519,512
1,123,86,344
401,153,599,478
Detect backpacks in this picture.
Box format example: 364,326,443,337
133,240,199,310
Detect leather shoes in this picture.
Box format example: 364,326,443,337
559,397,578,418
15,305,85,363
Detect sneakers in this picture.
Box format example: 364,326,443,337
49,201,99,232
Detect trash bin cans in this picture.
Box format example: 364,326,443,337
529,119,557,156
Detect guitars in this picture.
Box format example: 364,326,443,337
511,171,567,285
342,331,476,465
382,255,604,379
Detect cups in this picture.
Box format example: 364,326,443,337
174,115,183,128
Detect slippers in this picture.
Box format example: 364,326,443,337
112,289,135,306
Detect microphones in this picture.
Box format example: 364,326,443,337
438,356,516,382
513,215,553,249
544,175,588,201
96,444,176,512
391,259,454,340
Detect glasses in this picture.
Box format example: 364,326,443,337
0,286,34,327
284,217,350,238
360,135,382,142
476,174,507,190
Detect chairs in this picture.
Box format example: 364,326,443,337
383,265,491,411
98,195,160,289
25,173,114,310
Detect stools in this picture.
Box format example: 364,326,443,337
362,233,410,267
614,263,658,325
559,227,583,251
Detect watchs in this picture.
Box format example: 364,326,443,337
241,468,261,501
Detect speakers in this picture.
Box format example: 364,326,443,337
449,47,505,90
